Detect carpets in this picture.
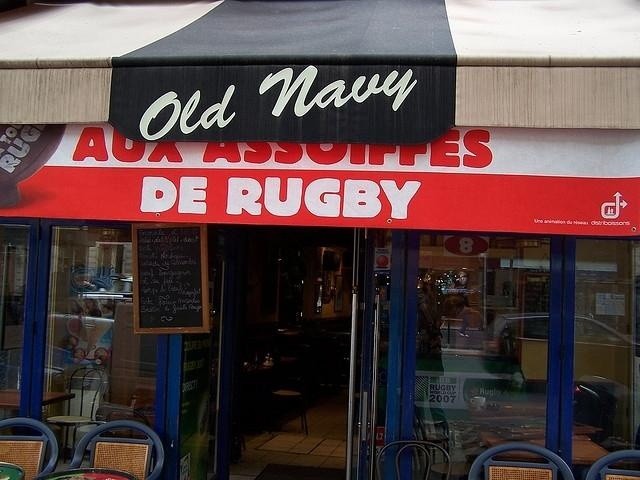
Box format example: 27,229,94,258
254,461,346,480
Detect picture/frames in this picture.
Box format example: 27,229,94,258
334,275,344,312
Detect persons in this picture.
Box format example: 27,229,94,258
414,283,451,444
439,294,486,350
81,298,102,317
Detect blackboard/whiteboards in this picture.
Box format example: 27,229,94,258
132,223,210,334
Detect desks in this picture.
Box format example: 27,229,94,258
477,414,612,467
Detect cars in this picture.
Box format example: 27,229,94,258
493,312,640,383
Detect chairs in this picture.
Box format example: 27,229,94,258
268,344,313,436
572,379,617,444
0,365,164,480
373,433,640,480
412,403,450,464
